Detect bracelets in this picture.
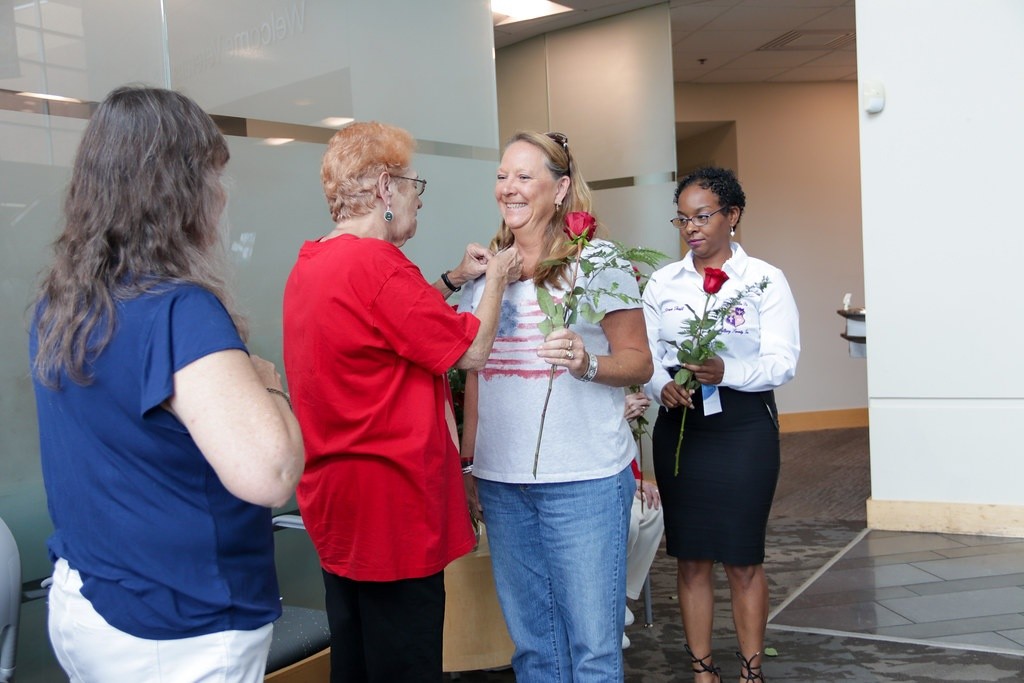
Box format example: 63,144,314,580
579,353,598,382
266,388,292,410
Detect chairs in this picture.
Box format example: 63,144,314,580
271,513,334,683
1,517,71,683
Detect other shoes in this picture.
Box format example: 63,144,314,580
624,606,634,626
622,632,630,649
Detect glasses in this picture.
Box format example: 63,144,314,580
390,174,426,196
546,131,571,178
671,207,725,230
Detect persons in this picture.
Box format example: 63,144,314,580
640,167,802,683
281,122,524,683
29,84,307,682
459,130,655,683
621,392,664,649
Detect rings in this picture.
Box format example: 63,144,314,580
566,350,574,359
642,406,646,410
568,340,573,350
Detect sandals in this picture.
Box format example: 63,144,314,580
683,644,722,683
735,651,764,683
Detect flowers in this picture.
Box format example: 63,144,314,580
655,267,771,476
533,211,674,481
630,267,654,515
